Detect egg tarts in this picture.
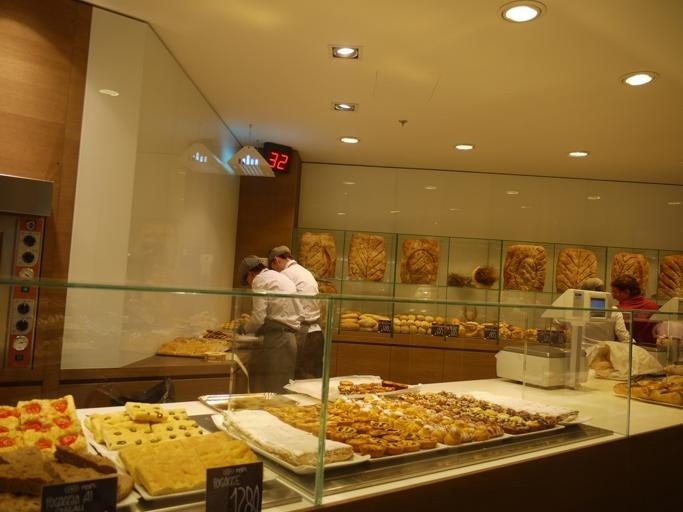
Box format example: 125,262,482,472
268,398,437,459
399,391,556,434
355,395,504,446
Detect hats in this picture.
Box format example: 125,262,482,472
582,277,604,291
238,256,261,285
268,245,290,269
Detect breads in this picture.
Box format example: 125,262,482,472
340,309,572,349
613,365,683,406
590,351,611,370
53,443,118,473
448,264,497,287
118,431,233,486
299,231,339,294
85,408,204,452
399,238,440,285
656,253,683,301
135,439,256,496
503,244,547,292
125,402,169,422
555,248,598,292
43,460,135,503
2,447,54,496
17,394,88,452
222,409,354,466
347,232,388,282
337,380,408,395
2,395,24,451
611,251,648,296
156,314,251,358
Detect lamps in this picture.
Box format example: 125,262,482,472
228,124,276,177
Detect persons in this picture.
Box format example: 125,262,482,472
268,245,324,380
581,278,637,344
232,254,304,394
610,274,661,348
